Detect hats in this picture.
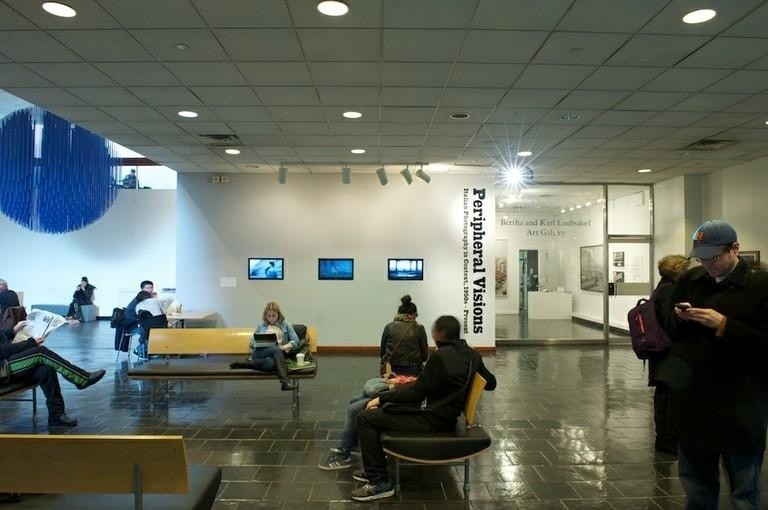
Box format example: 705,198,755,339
689,220,738,259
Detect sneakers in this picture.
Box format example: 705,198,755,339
350,469,371,484
317,453,353,471
281,383,298,391
229,361,247,368
351,480,395,502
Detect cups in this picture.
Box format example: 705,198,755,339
296,353,305,366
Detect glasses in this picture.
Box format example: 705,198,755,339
696,248,732,263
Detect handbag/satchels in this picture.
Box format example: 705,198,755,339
379,355,392,377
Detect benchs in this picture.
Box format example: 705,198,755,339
378,345,492,501
31,304,96,322
0,380,42,426
127,326,318,419
0,434,222,510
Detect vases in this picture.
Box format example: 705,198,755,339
580,244,603,292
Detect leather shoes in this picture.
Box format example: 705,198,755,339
47,412,78,426
73,368,106,390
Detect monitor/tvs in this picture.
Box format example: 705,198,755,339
248,257,285,280
388,258,424,282
317,258,354,280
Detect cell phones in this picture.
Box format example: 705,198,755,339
674,304,688,310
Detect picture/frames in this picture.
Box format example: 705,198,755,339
387,258,423,280
318,257,354,280
738,250,760,268
247,258,285,281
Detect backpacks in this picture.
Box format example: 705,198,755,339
627,282,676,360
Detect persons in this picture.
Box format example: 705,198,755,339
669,219,768,509
122,170,139,188
318,374,418,471
229,302,301,390
124,281,158,358
350,315,497,501
380,295,428,377
134,291,167,317
0,279,20,319
62,277,96,319
648,254,692,456
0,320,106,426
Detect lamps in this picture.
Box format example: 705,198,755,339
276,159,432,186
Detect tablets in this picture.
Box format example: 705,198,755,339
254,333,278,346
